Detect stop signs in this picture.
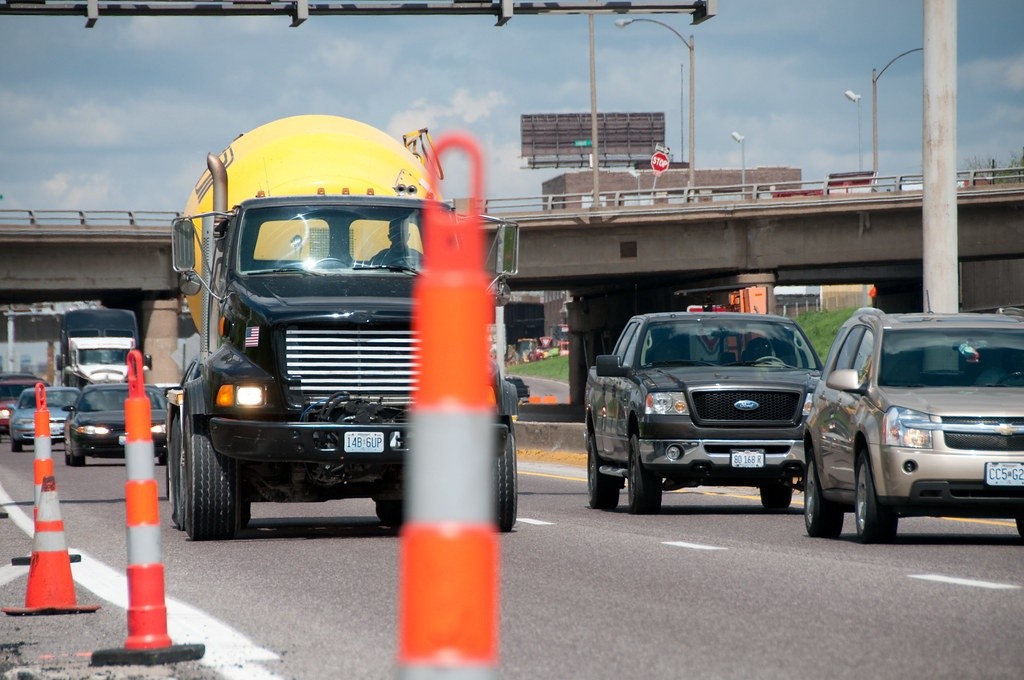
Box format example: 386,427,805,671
649,151,670,174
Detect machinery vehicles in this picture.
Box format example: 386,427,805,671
164,113,522,541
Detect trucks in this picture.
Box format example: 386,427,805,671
56,308,154,386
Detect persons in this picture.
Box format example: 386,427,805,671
369,218,423,269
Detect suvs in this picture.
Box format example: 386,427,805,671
584,311,826,516
801,305,1023,545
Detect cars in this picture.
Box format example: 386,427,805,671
63,384,167,468
1,373,80,454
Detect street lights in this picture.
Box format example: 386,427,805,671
730,130,747,198
613,16,696,196
840,88,864,172
